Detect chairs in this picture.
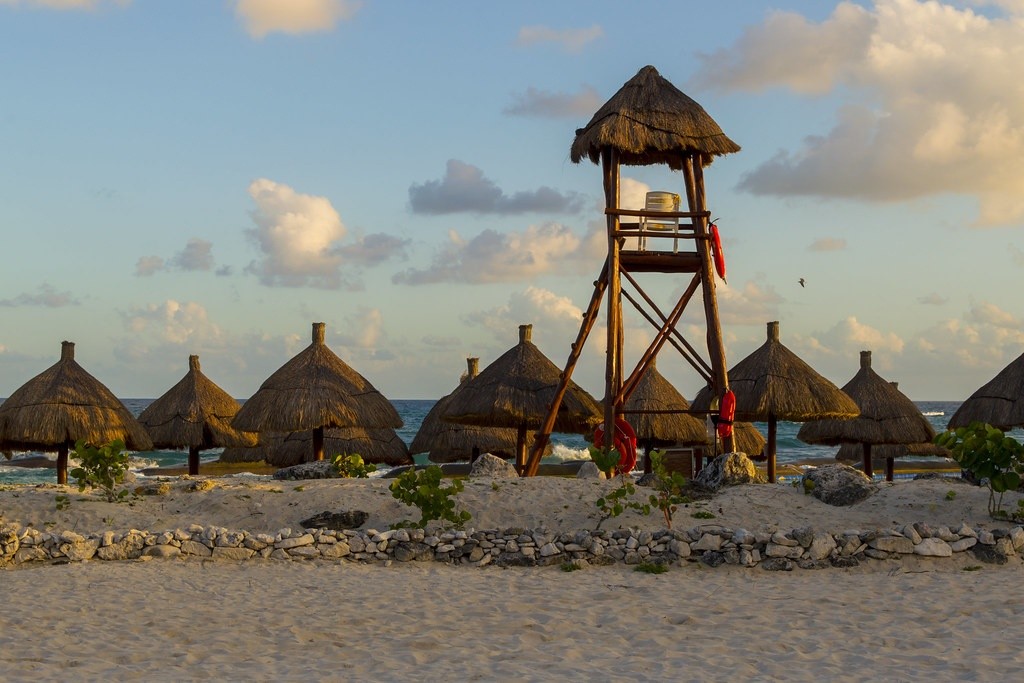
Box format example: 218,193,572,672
662,448,696,479
638,191,681,253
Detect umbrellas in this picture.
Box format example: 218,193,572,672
139,354,266,476
688,320,861,485
0,341,153,485
221,322,415,467
798,350,937,477
947,353,1024,447
409,323,604,476
585,354,708,478
836,381,953,482
701,420,770,460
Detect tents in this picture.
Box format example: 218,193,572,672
571,64,741,247
